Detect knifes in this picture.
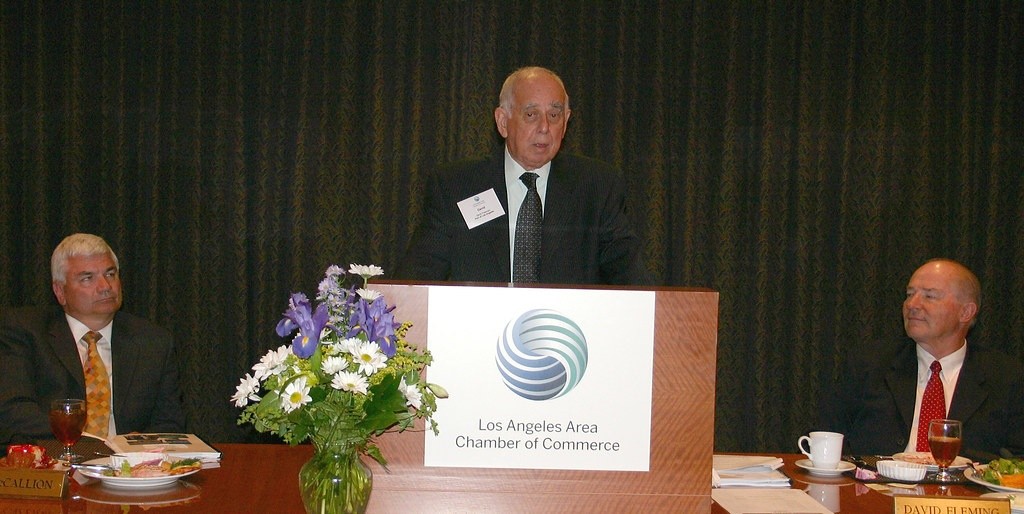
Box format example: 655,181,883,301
94,452,127,457
71,463,124,471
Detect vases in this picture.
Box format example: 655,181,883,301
299,441,373,514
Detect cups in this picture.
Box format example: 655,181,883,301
798,431,844,468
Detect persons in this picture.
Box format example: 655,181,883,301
839,259,1024,462
393,65,656,285
0,232,186,454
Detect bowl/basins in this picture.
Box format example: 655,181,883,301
875,460,927,481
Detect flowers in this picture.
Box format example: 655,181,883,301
229,263,450,514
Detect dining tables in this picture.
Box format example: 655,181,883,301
0,445,1024,514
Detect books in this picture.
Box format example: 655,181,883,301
713,454,791,487
105,434,222,463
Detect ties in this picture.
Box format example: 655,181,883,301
916,360,946,452
81,332,111,439
513,170,542,283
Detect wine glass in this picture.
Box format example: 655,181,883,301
928,418,962,484
49,399,86,462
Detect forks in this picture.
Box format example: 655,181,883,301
966,462,979,477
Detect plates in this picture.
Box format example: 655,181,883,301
979,492,1024,514
892,451,972,471
795,459,856,475
964,464,1024,493
77,455,202,486
